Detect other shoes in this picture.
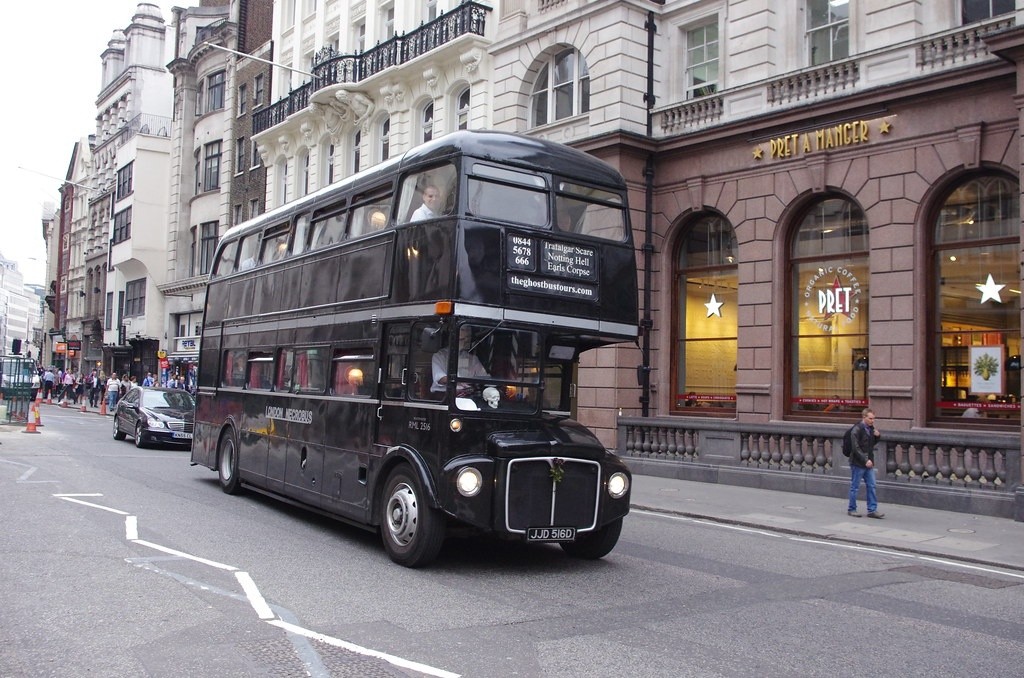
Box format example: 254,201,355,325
91,405,98,408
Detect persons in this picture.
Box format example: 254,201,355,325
232,353,246,388
848,408,886,518
33,359,196,412
410,184,442,223
429,325,492,400
825,395,844,412
961,395,985,417
686,392,700,407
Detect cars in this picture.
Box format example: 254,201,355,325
113,387,195,447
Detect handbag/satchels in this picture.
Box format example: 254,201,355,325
121,384,126,394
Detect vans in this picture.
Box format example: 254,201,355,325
0,356,41,400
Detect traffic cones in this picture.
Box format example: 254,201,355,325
36,389,40,401
17,396,27,421
45,389,52,405
77,397,88,412
0,392,3,405
37,389,44,402
62,391,69,408
98,398,108,415
35,400,44,426
22,404,40,434
10,396,18,416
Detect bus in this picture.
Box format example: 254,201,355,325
192,128,641,565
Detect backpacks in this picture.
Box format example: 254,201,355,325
844,423,858,457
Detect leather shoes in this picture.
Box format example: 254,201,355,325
867,511,885,518
848,512,862,517
